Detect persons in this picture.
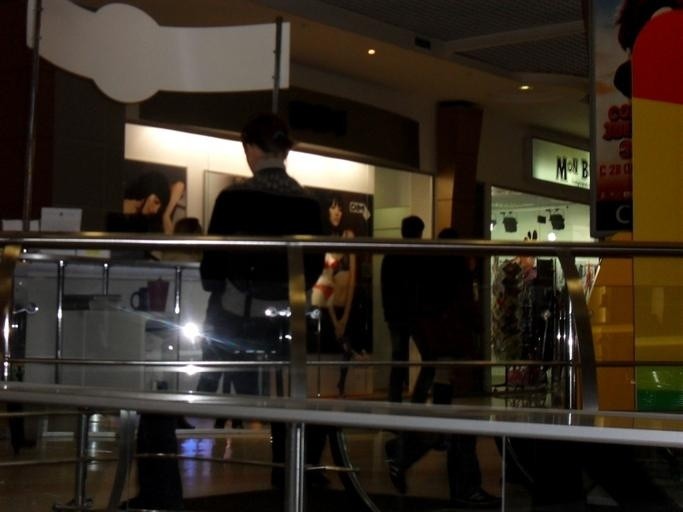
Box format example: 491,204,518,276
198,112,328,353
0,244,37,456
428,226,475,404
378,215,438,403
158,218,206,430
307,194,359,343
267,421,333,496
139,171,169,216
383,431,500,508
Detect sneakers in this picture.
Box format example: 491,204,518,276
385,439,412,495
452,487,504,506
122,490,184,509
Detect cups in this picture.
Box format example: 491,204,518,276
130,279,169,312
